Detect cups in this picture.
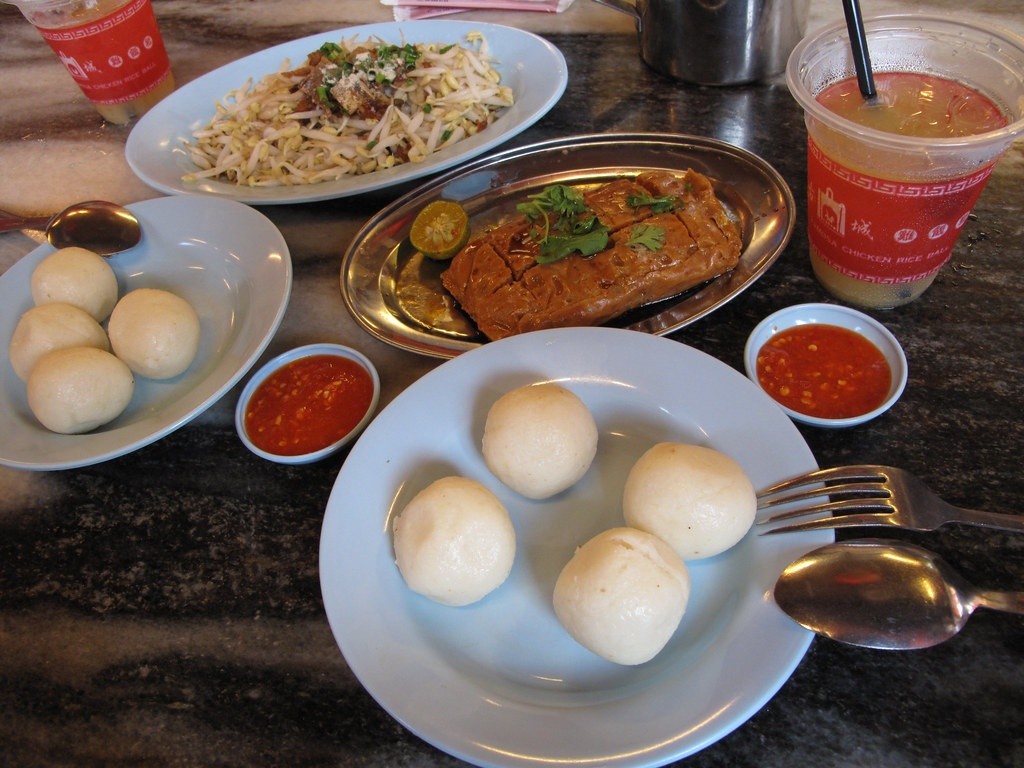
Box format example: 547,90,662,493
786,12,1023,310
0,1,175,125
594,0,811,85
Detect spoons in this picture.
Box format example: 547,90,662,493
0,201,143,255
774,539,1024,651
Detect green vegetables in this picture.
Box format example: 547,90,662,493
516,183,694,263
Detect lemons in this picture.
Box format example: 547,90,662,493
410,200,471,260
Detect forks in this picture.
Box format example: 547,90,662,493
756,464,1023,536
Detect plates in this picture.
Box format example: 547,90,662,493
339,132,797,361
0,196,294,472
743,303,908,427
235,341,380,464
318,327,835,768
124,20,569,205
